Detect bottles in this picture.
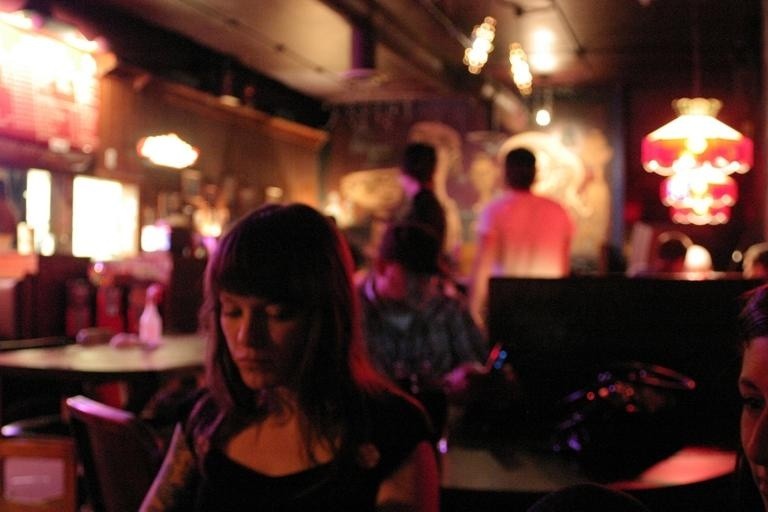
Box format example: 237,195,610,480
137,285,162,351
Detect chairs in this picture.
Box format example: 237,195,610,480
62,394,164,510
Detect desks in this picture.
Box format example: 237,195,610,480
435,441,738,512
0,333,206,438
